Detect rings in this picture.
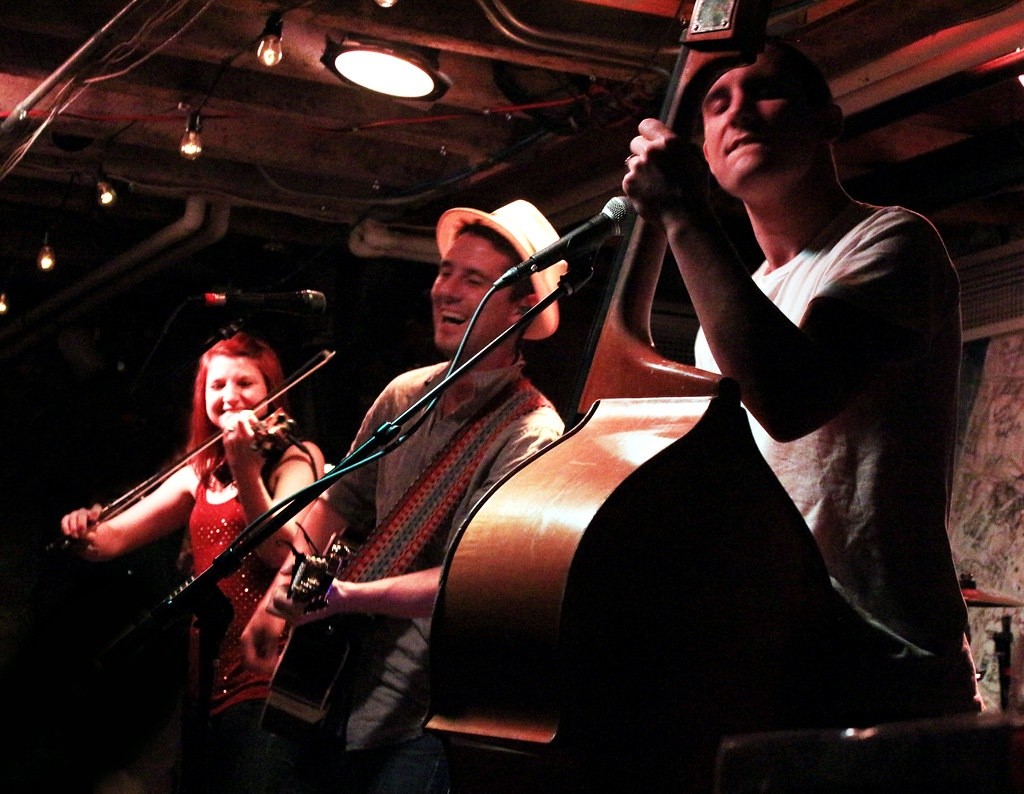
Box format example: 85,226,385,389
626,153,639,162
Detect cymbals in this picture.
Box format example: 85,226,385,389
960,587,1024,609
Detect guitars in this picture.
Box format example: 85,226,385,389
259,527,358,741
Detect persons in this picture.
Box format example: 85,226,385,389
59,333,325,794
239,197,573,794
618,32,983,721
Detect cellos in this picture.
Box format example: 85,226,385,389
418,0,838,794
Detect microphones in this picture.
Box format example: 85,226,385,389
493,197,637,290
202,290,326,317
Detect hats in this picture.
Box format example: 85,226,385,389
436,199,567,340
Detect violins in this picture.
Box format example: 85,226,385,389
211,407,299,487
